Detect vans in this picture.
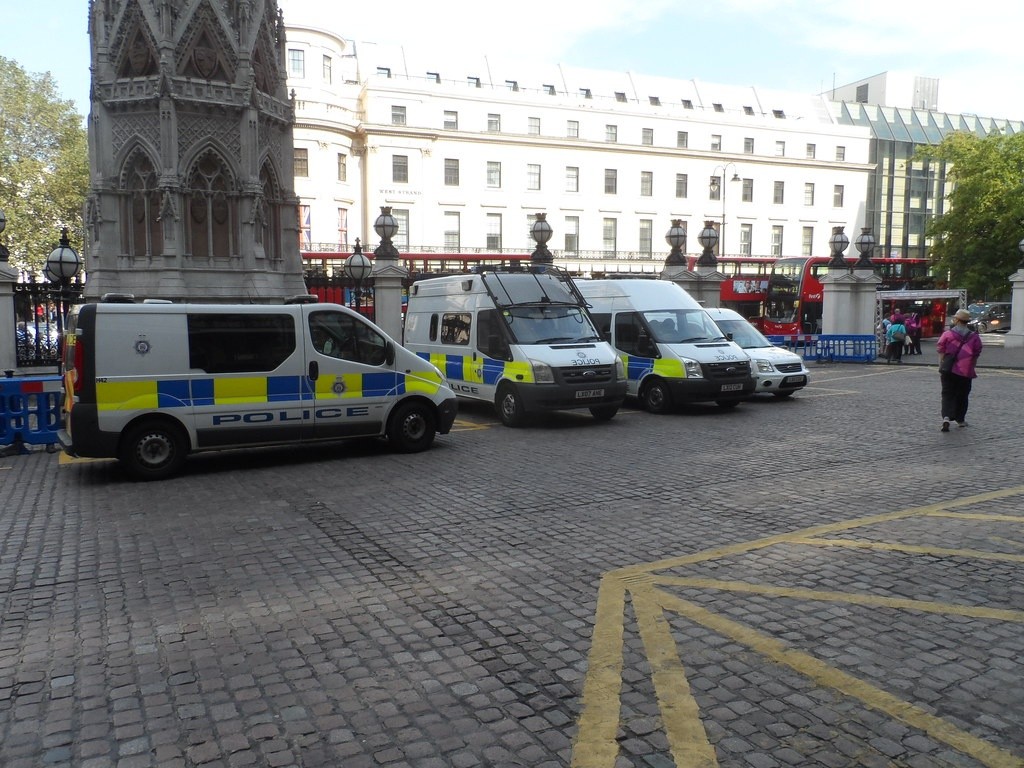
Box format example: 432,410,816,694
54,291,461,483
543,277,758,415
402,261,629,429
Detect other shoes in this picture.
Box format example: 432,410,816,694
958,421,968,427
897,360,902,363
941,416,950,431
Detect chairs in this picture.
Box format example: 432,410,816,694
542,318,555,328
519,316,536,328
650,319,675,329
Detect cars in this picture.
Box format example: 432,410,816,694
967,301,1011,334
17,320,59,360
642,306,811,401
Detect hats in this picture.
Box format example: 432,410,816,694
952,309,972,321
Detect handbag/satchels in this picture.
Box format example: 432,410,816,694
904,335,912,346
892,331,904,341
939,353,955,376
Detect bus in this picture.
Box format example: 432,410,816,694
757,256,951,347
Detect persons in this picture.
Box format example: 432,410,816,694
739,280,761,293
18,303,56,358
877,308,922,365
937,309,982,432
